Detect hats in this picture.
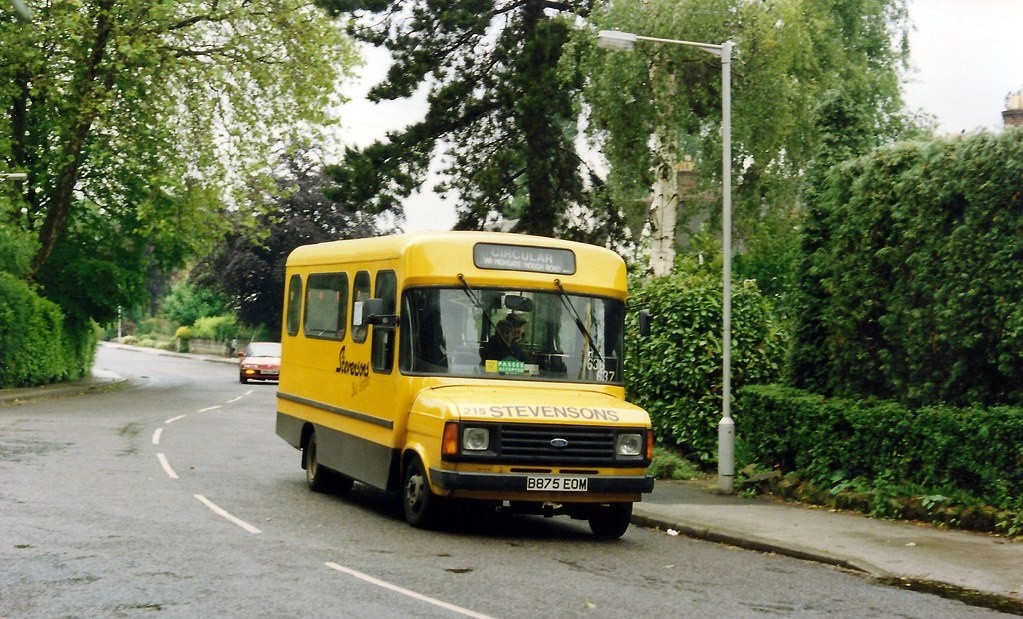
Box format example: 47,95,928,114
507,313,528,326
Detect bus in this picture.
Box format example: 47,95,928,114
275,229,654,543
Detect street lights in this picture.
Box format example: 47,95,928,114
594,30,737,495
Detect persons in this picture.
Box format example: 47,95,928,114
481,313,527,368
229,338,237,357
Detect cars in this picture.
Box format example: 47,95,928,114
237,341,282,385
